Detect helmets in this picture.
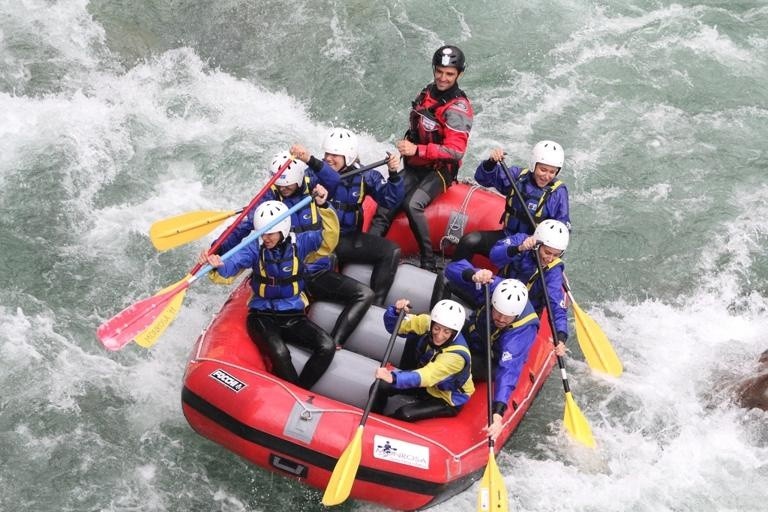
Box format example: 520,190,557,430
253,200,292,243
529,139,564,178
491,278,528,318
533,219,570,257
269,152,307,188
427,299,466,342
321,127,358,167
432,45,466,73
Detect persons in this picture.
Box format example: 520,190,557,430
367,297,477,422
195,142,377,351
429,219,570,357
451,140,572,266
442,258,540,445
206,183,342,391
365,45,475,272
303,126,406,307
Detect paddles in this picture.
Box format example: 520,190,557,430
97,190,319,351
149,158,388,251
322,303,413,506
477,278,509,512
132,154,298,348
499,152,623,378
533,241,598,450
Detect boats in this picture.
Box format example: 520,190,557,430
181,182,560,507
